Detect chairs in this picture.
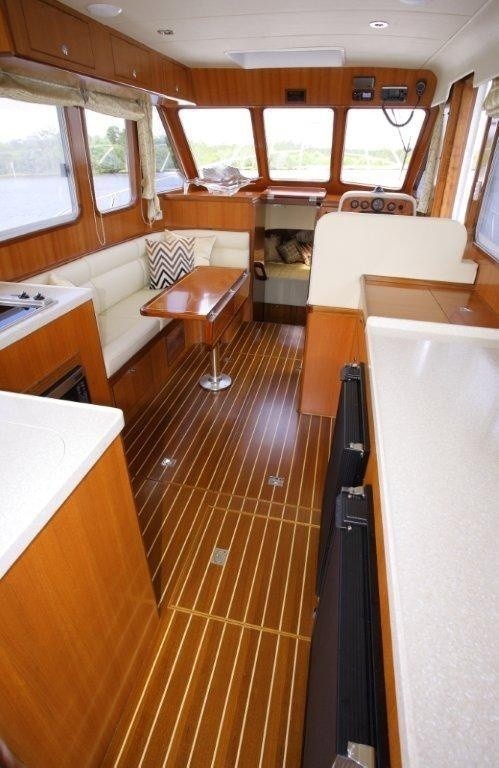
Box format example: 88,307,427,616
309,184,479,315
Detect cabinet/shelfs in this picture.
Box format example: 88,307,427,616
113,355,154,414
165,321,185,365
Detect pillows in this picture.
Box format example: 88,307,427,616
264,230,313,266
145,229,215,291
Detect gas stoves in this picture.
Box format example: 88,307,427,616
0,292,53,332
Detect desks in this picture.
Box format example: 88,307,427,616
141,265,248,391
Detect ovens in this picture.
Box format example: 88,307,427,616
41,365,96,406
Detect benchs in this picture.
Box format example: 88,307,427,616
12,228,254,379
